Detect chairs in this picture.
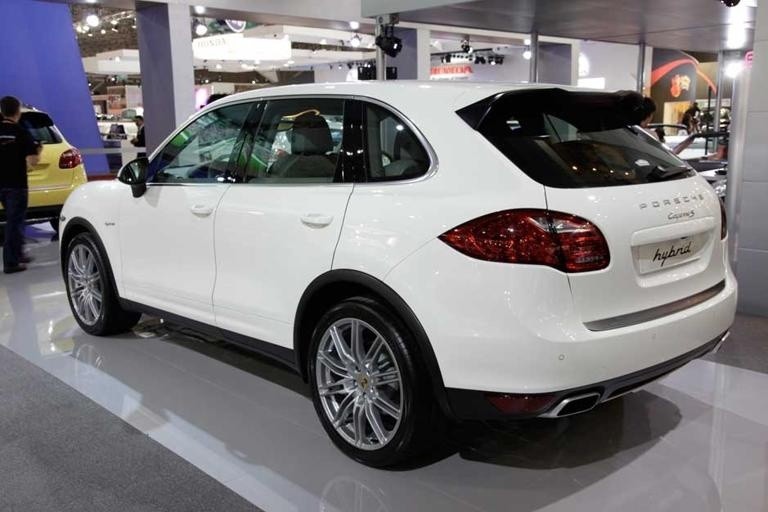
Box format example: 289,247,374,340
384,128,430,178
266,114,339,178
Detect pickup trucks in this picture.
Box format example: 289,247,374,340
93,104,143,134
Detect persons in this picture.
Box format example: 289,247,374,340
0,96,39,273
117,125,127,141
107,124,117,138
635,96,659,141
131,116,145,158
683,102,713,136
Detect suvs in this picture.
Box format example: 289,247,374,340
57,79,740,470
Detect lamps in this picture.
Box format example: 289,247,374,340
431,39,504,66
74,14,137,37
376,14,403,57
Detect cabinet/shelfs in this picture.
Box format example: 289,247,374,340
106,84,143,117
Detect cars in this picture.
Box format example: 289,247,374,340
0,101,90,242
95,111,114,120
69,328,634,512
697,106,731,132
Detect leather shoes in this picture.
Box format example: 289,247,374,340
4,257,31,273
